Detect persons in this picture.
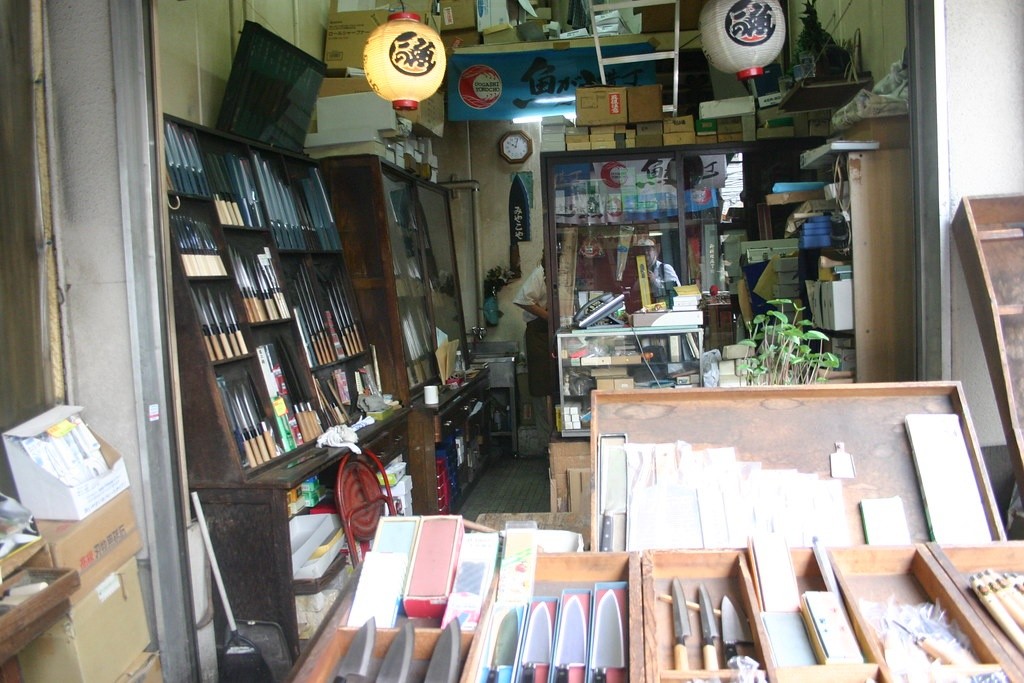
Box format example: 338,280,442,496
513,248,559,479
637,239,682,299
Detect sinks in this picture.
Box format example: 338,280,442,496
473,352,513,358
472,358,512,362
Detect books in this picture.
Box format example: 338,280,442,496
669,284,702,311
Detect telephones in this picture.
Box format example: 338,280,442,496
573,292,624,328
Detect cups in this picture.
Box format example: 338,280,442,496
423,385,439,405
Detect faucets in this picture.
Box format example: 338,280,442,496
473,327,487,340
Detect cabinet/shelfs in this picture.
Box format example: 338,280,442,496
828,149,918,383
162,113,416,660
535,136,828,435
316,154,495,517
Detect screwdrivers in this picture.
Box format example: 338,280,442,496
892,619,954,665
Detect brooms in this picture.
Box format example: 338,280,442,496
191,491,273,683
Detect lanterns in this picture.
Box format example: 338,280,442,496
698,0,786,81
362,12,447,112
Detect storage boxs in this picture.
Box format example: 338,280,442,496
289,379,1024,683
439,0,475,30
542,10,630,40
441,28,479,48
425,14,442,33
1,555,163,683
564,401,582,430
562,355,642,366
36,489,144,605
574,85,628,128
526,7,552,20
540,96,832,152
595,376,635,390
483,26,523,44
632,311,703,327
740,181,855,331
2,404,130,521
716,337,856,388
628,84,664,124
324,0,389,70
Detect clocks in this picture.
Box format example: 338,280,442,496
499,130,533,164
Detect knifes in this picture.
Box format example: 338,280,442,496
163,127,432,466
335,578,748,683
599,445,626,553
509,172,531,278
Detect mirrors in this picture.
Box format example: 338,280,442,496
381,162,466,396
